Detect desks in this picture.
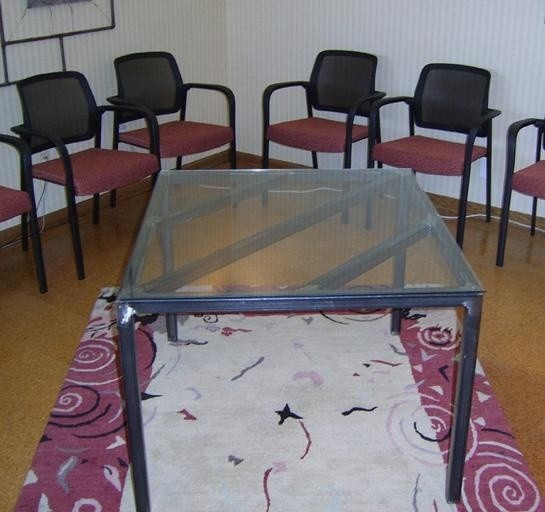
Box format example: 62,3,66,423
114,168,487,512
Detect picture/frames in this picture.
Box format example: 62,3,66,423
0,0,116,45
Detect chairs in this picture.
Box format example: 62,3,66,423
261,50,383,168
106,51,236,207
496,118,544,268
367,64,501,250
0,134,51,294
0,71,162,253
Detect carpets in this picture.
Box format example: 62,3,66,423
13,286,544,512
